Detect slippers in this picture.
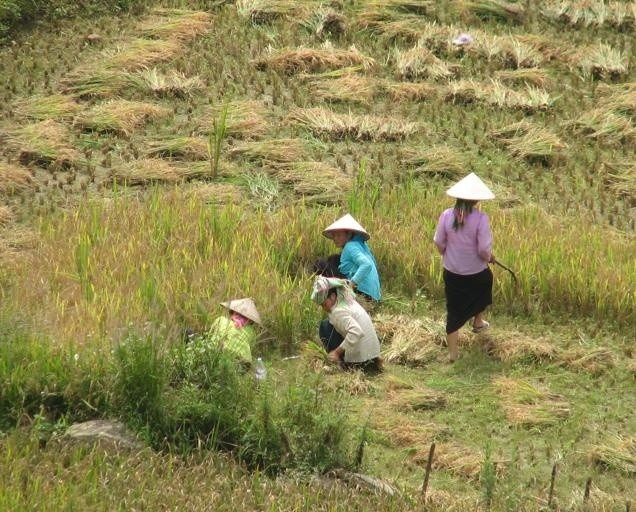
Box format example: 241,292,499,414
473,320,489,332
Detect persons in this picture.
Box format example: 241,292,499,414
314,212,381,303
434,172,494,361
309,275,383,372
183,295,262,375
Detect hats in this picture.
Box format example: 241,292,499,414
220,298,260,324
446,172,495,200
322,213,370,241
